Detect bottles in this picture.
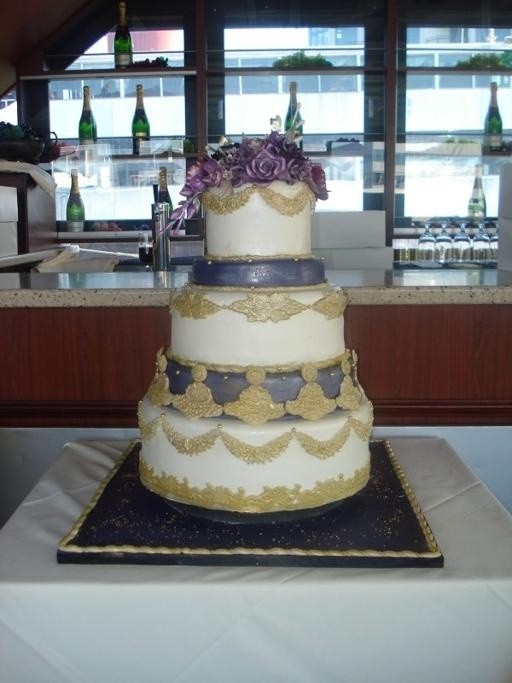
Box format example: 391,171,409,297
151,201,171,272
79,85,97,160
131,82,151,155
467,163,487,225
481,82,503,152
283,81,303,152
114,0,132,71
65,168,84,233
158,166,173,216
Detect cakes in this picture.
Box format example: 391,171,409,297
135,179,374,513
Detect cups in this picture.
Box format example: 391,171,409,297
136,230,153,272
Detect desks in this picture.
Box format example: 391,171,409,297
0,435,511,683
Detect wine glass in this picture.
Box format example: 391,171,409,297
414,222,498,265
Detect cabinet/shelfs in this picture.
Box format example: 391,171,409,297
0,0,512,270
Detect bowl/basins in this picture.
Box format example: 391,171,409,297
0,135,61,167
